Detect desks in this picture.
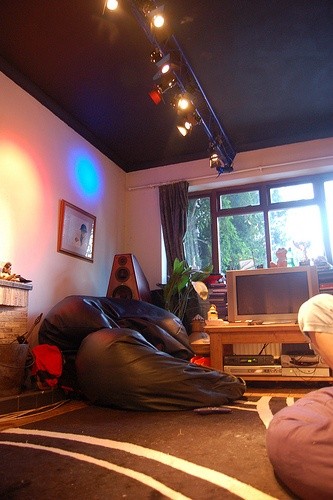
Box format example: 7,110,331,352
0,280,34,344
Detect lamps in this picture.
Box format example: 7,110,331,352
98,0,241,178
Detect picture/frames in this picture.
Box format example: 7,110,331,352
56,198,97,264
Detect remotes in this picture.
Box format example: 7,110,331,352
195,408,231,415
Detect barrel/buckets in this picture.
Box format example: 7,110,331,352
0,344,35,396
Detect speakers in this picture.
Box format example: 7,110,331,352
107,253,151,304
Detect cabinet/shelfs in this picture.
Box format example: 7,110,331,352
200,319,333,400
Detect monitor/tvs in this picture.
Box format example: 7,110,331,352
226,265,319,321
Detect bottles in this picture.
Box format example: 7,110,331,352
286,248,293,267
207,304,218,320
